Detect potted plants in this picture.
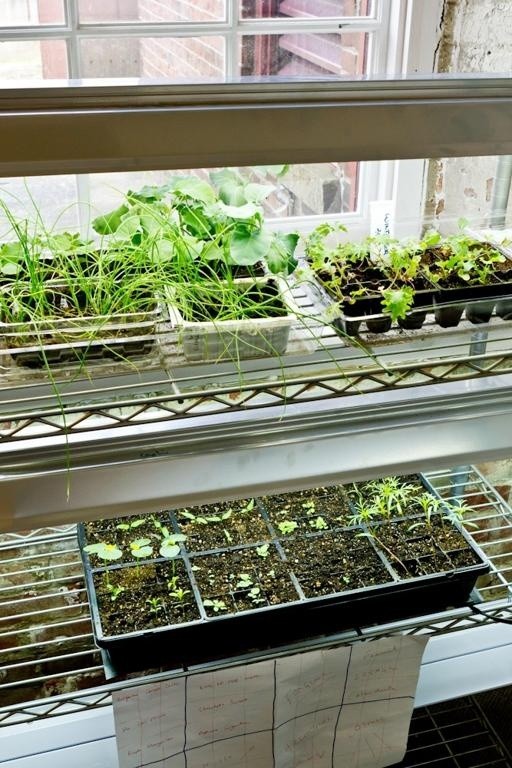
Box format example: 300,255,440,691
0,177,170,368
95,182,394,381
76,469,493,672
303,250,439,338
410,238,512,328
145,184,270,286
